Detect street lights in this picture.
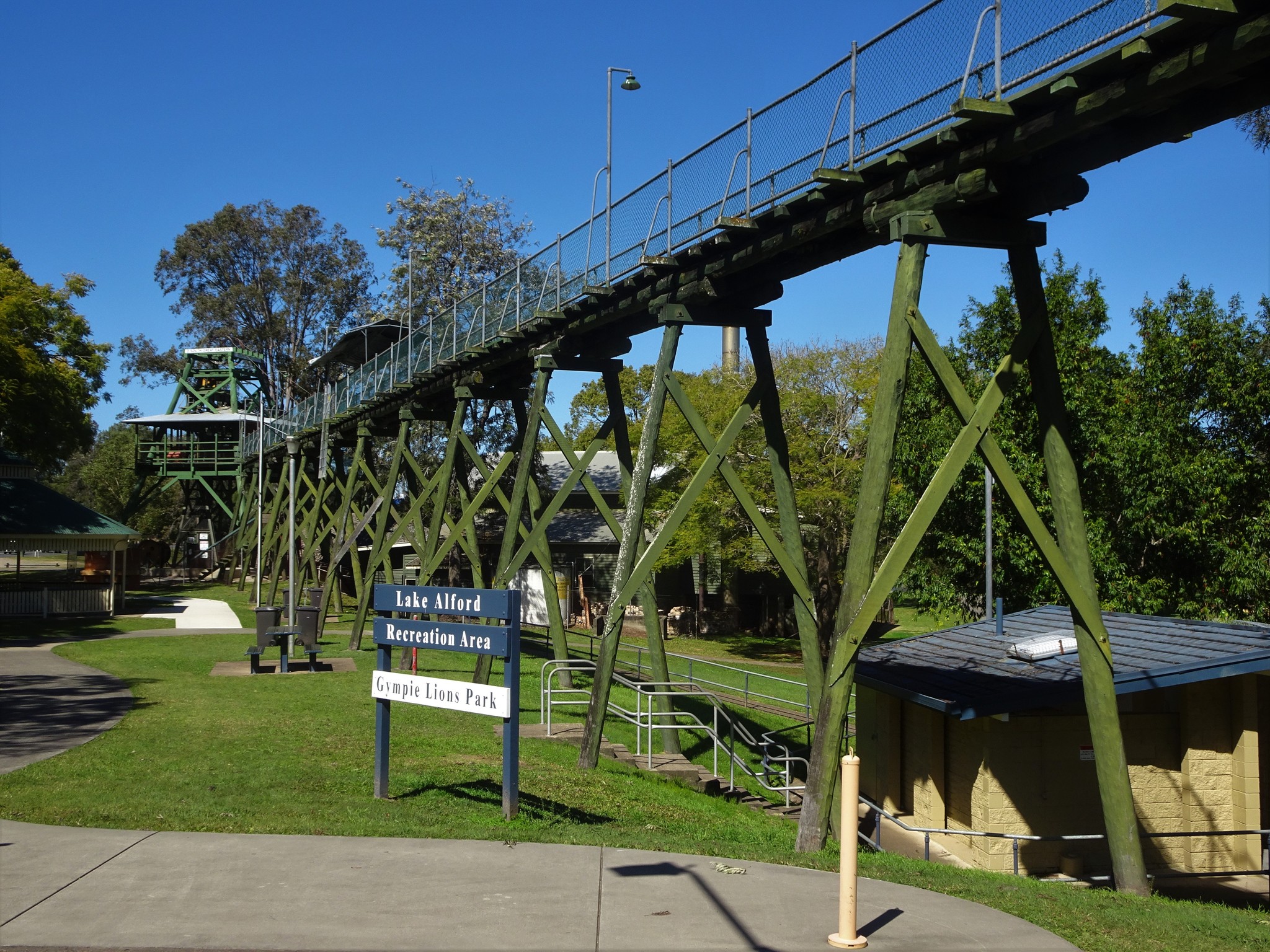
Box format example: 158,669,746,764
407,249,432,384
323,326,342,420
242,398,255,461
274,370,289,443
286,435,302,657
604,67,641,288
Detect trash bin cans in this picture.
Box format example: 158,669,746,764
252,606,286,647
295,606,322,646
281,588,290,618
308,588,324,606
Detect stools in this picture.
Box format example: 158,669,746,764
304,644,323,673
244,646,266,675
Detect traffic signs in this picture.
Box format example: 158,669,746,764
375,583,512,620
374,618,510,656
371,670,511,718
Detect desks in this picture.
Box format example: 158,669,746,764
265,626,303,673
